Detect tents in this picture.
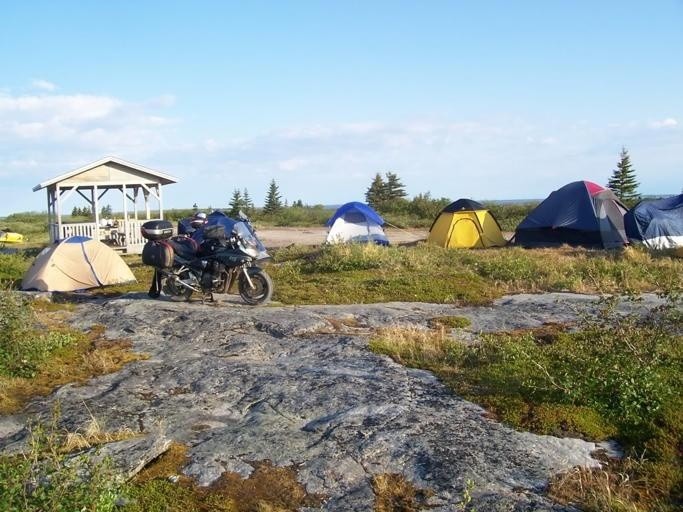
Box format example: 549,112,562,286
425,199,516,249
516,181,632,249
326,202,391,247
621,195,683,251
20,235,139,294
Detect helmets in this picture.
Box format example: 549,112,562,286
190,212,207,228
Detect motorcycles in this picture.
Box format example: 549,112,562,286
140,210,273,306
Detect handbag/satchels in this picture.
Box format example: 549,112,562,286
142,239,175,268
